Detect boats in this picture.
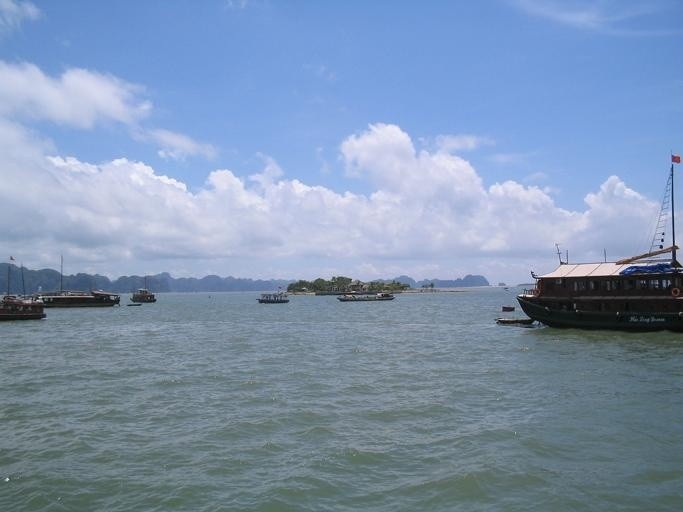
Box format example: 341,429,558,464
336,292,396,301
0,255,47,321
256,291,290,304
493,317,535,325
127,303,142,306
31,255,120,308
516,149,683,332
130,272,157,303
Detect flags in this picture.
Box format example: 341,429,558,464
279,287,281,288
672,155,680,163
11,256,16,260
